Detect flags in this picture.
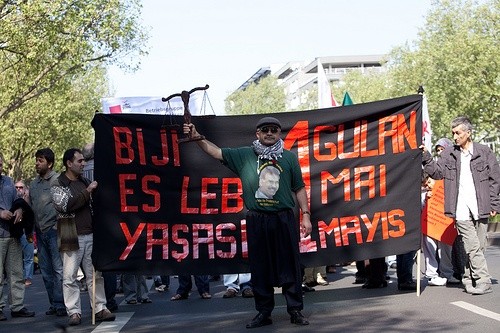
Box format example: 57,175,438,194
422,93,432,155
317,58,338,108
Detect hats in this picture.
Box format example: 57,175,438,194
256,117,281,129
435,138,453,150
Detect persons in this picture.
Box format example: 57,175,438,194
49,148,116,326
0,158,35,322
182,117,312,328
419,116,500,295
0,116,500,329
27,148,68,317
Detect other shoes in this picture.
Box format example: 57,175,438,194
301,259,493,295
243,287,254,297
202,292,211,298
170,294,182,301
46,310,56,315
224,290,235,297
25,280,32,286
56,308,66,316
127,300,137,304
142,299,152,303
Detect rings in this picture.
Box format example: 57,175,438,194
20,219,22,222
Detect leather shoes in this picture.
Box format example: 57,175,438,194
290,311,309,325
246,313,272,329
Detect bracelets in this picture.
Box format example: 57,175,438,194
302,210,311,216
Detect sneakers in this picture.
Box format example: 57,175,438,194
69,313,81,325
11,307,35,317
0,311,8,321
95,310,116,321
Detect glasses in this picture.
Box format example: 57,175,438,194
260,127,281,133
15,186,24,189
